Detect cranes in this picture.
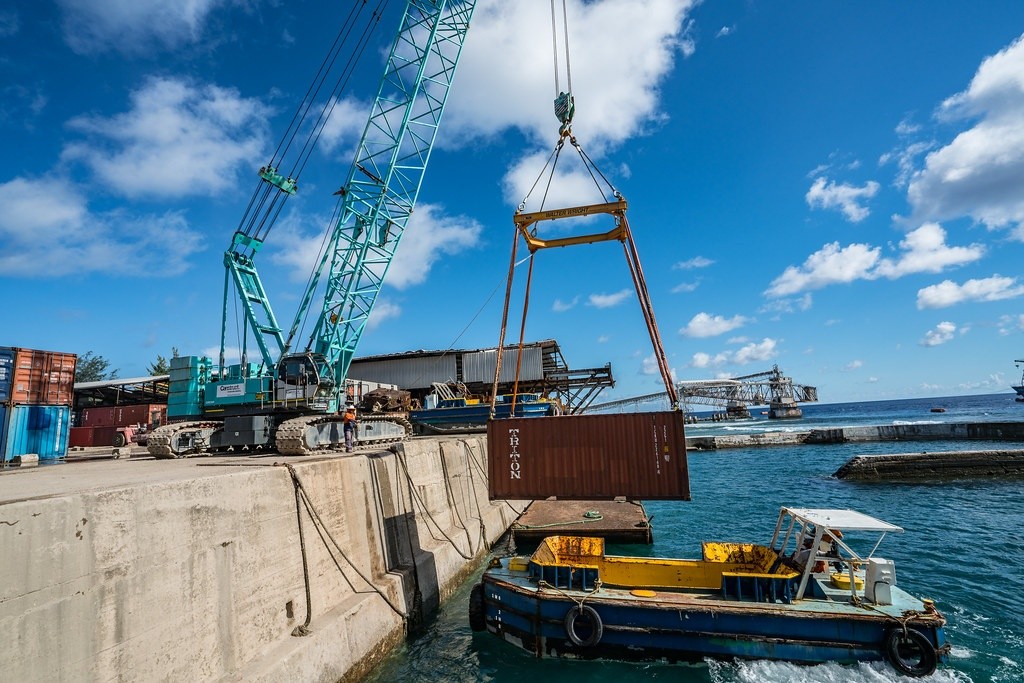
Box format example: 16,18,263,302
671,378,818,419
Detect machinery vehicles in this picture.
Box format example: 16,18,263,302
139,0,619,459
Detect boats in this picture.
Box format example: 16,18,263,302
469,504,953,676
1012,359,1024,402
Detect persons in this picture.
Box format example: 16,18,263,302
344,405,357,453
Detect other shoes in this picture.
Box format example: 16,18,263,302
346,450,352,453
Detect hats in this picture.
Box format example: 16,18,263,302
347,405,355,409
